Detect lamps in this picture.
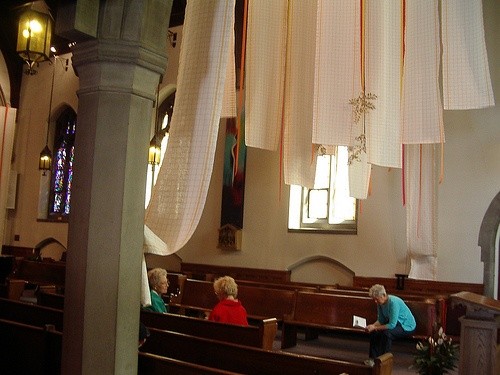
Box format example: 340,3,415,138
39,68,56,177
14,0,56,76
148,84,161,165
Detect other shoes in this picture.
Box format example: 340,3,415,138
363,359,374,367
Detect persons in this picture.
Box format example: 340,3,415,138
143,268,169,313
363,284,416,367
209,276,249,327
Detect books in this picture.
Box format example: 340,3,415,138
352,315,367,329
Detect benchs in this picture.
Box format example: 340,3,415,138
0,260,448,375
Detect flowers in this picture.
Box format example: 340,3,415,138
413,326,459,375
